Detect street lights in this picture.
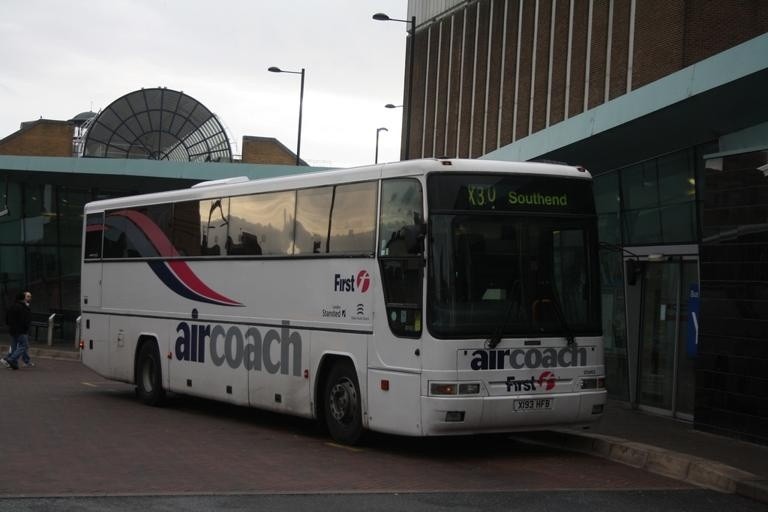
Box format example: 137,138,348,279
371,10,417,159
267,61,309,165
371,123,389,163
383,100,407,111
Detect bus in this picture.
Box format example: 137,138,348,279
70,152,612,450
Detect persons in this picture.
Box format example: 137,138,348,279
6,292,31,370
0,291,36,368
